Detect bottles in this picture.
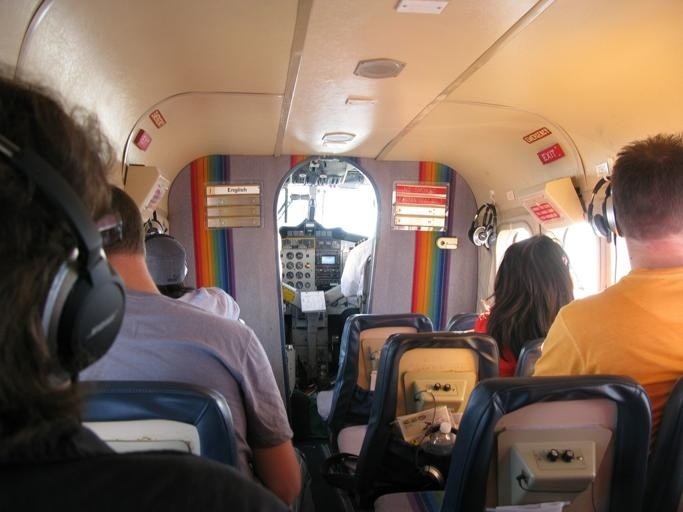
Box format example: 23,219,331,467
424,421,457,455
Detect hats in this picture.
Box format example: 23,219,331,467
140,235,190,287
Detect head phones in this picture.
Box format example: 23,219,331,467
0,135,126,372
468,203,498,248
588,176,624,239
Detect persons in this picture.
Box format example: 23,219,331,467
142,233,241,321
473,231,573,379
528,132,683,445
338,237,377,298
0,66,306,510
74,182,303,506
